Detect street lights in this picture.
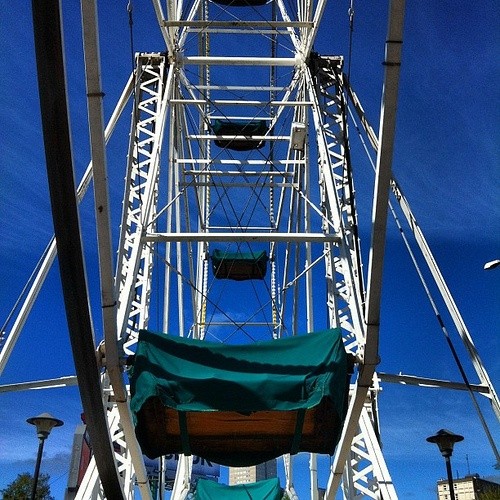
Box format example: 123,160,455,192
426,429,464,500
26,412,64,500
